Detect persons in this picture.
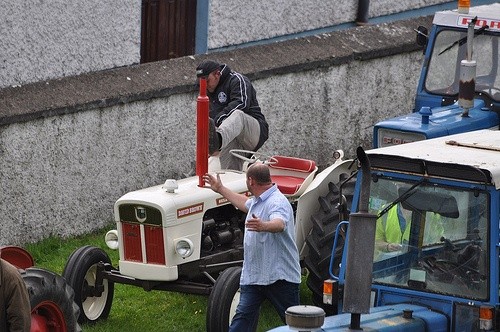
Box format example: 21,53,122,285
195,60,269,171
375,196,445,255
202,162,302,331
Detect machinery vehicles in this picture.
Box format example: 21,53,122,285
0,0,500,332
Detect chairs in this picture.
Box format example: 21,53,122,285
264,155,318,198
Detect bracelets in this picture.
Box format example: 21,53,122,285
387,242,390,251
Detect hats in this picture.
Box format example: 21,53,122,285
197,60,221,76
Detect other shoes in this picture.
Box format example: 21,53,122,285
210,117,217,154
